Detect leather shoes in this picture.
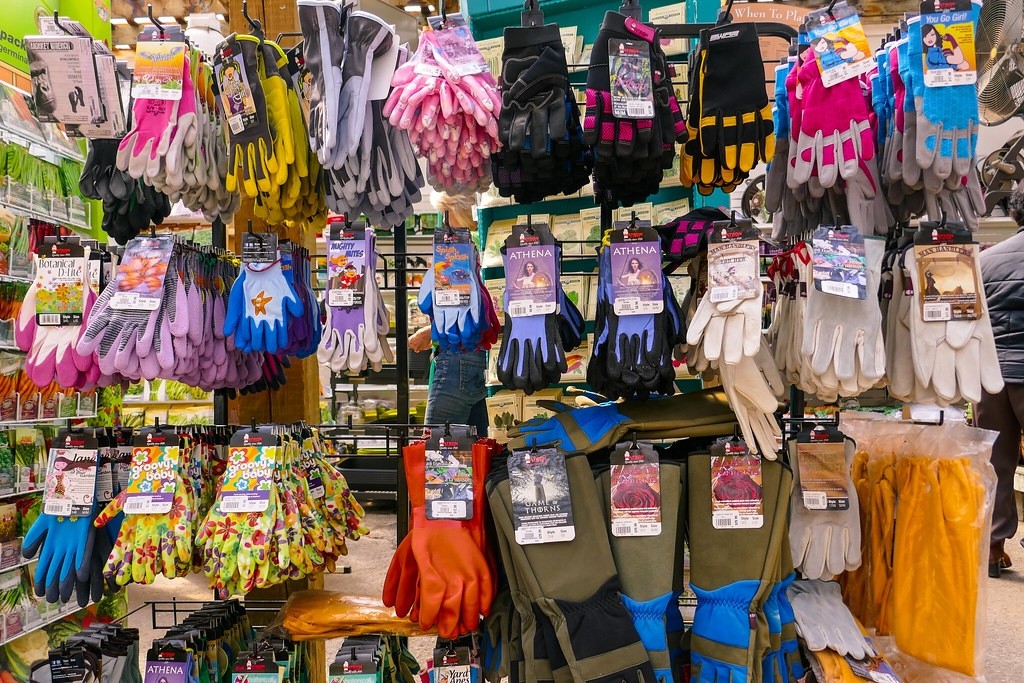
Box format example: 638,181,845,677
987,550,1012,577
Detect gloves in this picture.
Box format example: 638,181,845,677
766,0,991,242
13,234,317,401
319,224,397,375
266,430,989,683
19,422,420,683
67,85,85,113
418,217,1004,461
24,0,776,246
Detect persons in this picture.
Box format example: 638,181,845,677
978,178,1024,577
511,262,538,290
616,257,643,287
408,189,489,439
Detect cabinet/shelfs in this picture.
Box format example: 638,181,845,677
1,0,132,683
